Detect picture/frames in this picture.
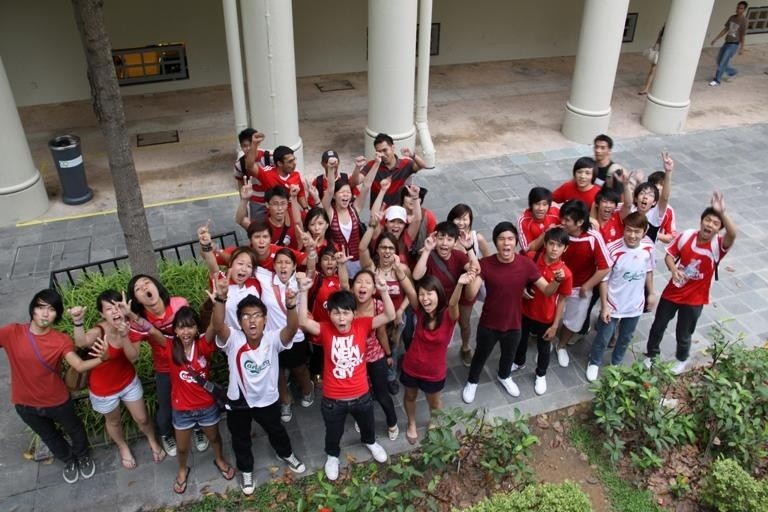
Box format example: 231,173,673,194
745,6,768,34
622,12,639,43
417,23,440,57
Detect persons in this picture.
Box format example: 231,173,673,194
112,129,490,496
1,291,111,483
511,134,736,396
66,290,168,470
462,222,565,403
709,0,748,86
638,23,664,95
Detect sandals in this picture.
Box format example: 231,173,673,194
636,88,650,96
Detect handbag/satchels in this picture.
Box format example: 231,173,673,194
360,224,373,257
76,347,94,365
211,379,226,410
641,46,659,64
477,281,486,302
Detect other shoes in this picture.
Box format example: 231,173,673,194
389,424,399,443
355,423,360,432
405,426,418,445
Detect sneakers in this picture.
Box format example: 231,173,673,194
710,70,738,88
239,471,255,495
366,442,387,463
161,434,179,458
324,455,339,481
77,454,96,479
194,429,209,453
389,377,401,394
276,451,306,473
280,382,320,422
462,328,692,403
62,459,78,483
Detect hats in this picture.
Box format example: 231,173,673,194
383,205,410,228
320,149,340,163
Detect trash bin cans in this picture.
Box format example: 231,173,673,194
48,134,94,205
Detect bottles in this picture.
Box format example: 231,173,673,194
672,257,703,288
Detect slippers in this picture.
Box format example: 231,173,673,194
118,447,137,470
174,466,190,494
149,443,166,464
213,457,235,481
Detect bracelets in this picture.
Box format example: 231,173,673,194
72,322,83,327
